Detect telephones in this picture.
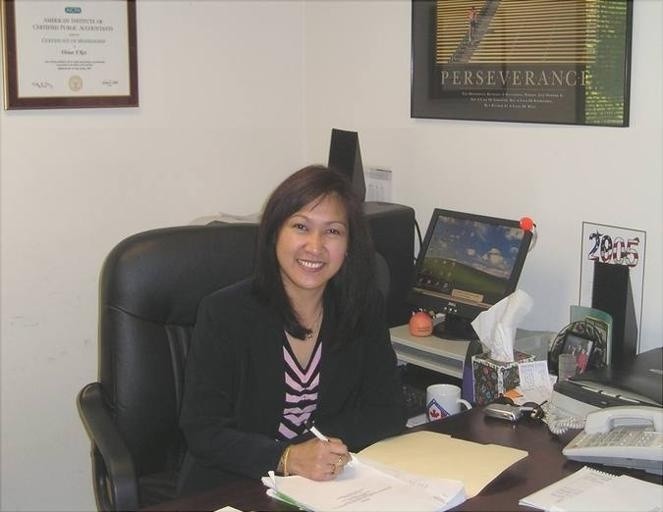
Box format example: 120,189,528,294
561,405,663,476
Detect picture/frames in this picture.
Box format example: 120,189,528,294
409,0,634,129
0,1,141,111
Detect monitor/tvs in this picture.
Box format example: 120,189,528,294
403,207,534,341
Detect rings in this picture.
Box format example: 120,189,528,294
335,454,344,466
330,463,336,474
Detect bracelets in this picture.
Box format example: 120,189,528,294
281,444,290,476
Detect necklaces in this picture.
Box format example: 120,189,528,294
288,308,324,340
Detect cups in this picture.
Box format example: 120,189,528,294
426,384,472,423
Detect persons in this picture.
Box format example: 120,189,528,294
564,341,589,374
169,164,409,499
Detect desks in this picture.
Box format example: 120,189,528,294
140,346,662,512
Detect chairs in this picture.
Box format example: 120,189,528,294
76,219,264,512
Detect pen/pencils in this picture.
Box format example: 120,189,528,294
303,420,353,469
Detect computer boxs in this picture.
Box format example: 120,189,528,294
360,199,415,328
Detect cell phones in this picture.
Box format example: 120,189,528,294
483,402,534,425
